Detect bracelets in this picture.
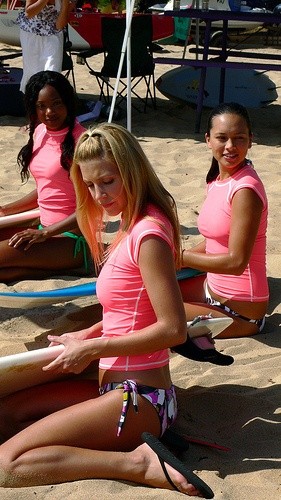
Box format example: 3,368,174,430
182,249,186,265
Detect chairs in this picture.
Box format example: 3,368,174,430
58,22,75,90
77,15,159,120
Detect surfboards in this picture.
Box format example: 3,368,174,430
0,266,207,309
154,65,278,110
0,317,234,396
0,210,40,228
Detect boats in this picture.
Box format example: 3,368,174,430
144,0,277,37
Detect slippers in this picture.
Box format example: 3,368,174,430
141,431,214,499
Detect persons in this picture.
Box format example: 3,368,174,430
16,0,69,95
175,102,270,339
0,70,95,281
0,124,214,500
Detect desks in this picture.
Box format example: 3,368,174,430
154,7,281,134
